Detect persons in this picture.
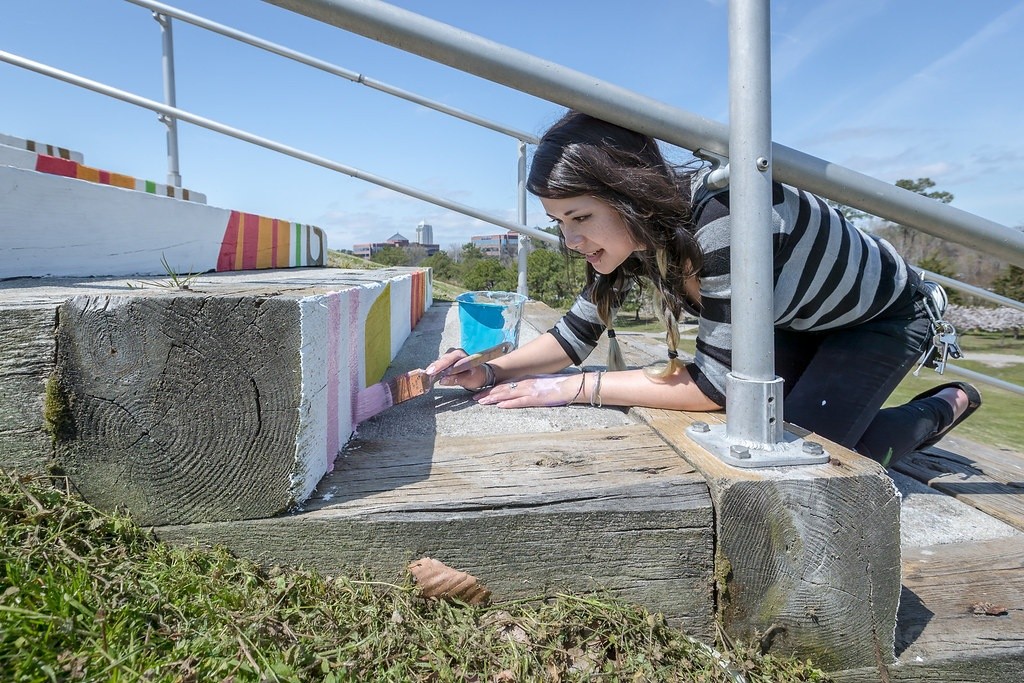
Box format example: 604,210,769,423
426,107,982,471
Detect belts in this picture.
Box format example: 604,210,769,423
882,268,920,318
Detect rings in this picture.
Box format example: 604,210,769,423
510,382,517,389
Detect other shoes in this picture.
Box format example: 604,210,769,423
909,381,983,450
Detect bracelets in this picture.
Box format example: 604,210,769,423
565,371,585,407
466,363,496,393
590,370,606,408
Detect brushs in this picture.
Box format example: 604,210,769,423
355,341,515,425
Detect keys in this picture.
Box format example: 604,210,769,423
913,324,964,376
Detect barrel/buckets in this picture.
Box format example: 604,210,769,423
455,291,528,356
